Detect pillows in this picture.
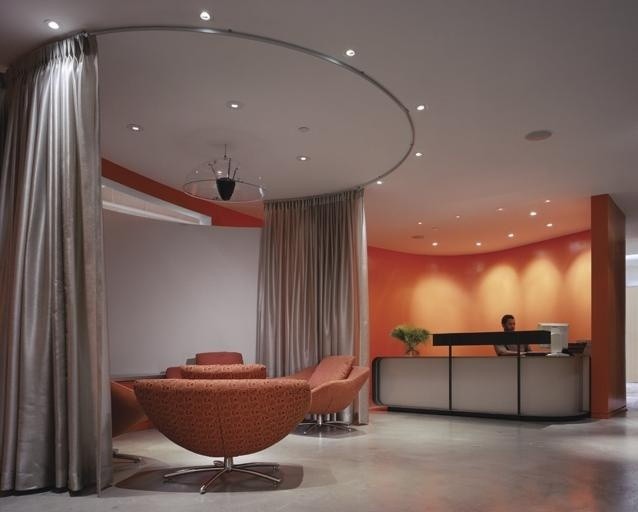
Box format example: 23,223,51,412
180,364,268,379
307,357,356,391
196,353,244,364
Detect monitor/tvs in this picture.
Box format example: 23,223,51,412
538,323,570,358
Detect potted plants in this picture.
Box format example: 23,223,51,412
391,326,432,356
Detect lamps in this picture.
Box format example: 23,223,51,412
182,144,268,204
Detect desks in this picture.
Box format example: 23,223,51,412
111,375,169,433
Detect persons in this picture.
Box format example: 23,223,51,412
493,314,533,356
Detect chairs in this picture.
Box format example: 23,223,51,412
290,355,371,436
166,366,183,380
136,363,312,494
110,380,152,463
